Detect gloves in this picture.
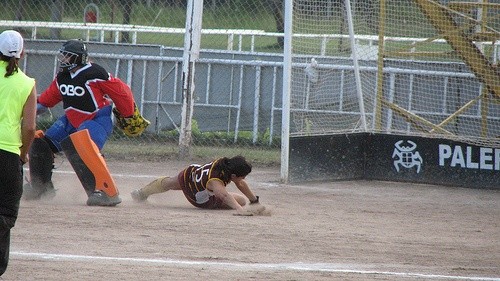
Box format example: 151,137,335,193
250,196,259,204
113,106,150,137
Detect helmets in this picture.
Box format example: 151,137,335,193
57,38,88,71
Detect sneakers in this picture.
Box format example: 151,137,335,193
19,181,56,202
130,189,146,202
88,189,121,205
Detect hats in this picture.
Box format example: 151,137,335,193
0,30,24,60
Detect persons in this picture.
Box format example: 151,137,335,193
0,30,37,276
22,38,150,206
130,155,261,216
